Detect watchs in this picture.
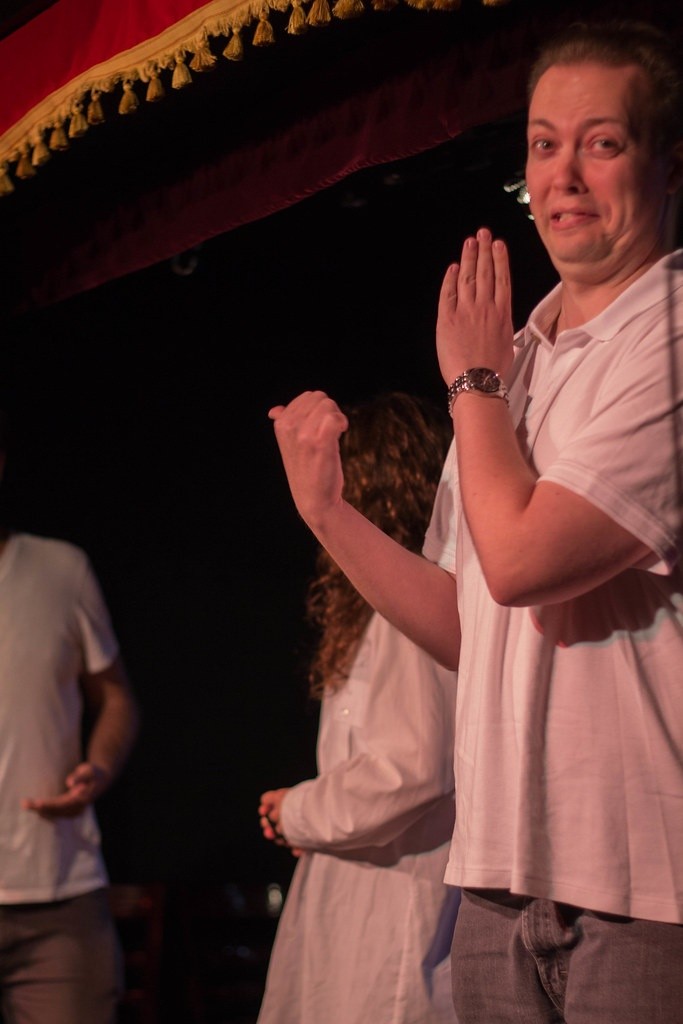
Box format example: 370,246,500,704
447,367,511,418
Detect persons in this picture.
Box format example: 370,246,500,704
0,533,144,1024
257,392,459,1024
267,24,683,1024
